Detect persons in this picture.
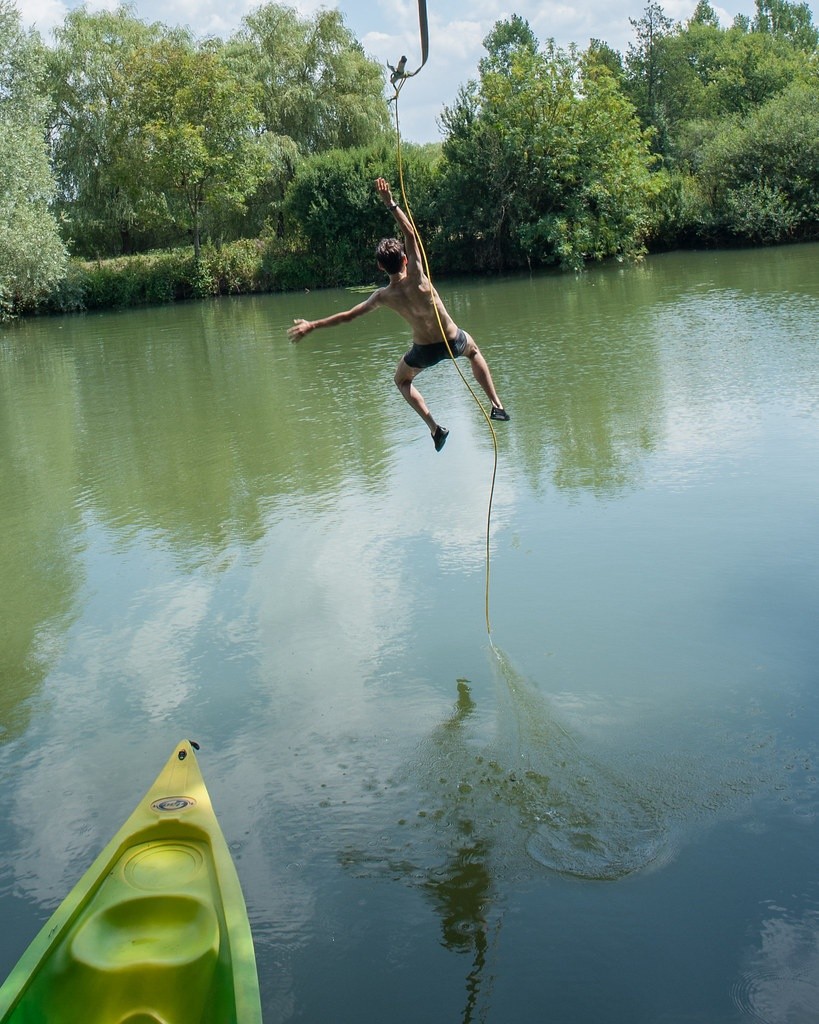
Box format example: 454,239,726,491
288,178,510,453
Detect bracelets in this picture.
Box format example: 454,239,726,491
386,204,398,212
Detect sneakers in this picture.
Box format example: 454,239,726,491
431,425,449,452
490,407,510,421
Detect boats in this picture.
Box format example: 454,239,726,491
2,738,264,1024
